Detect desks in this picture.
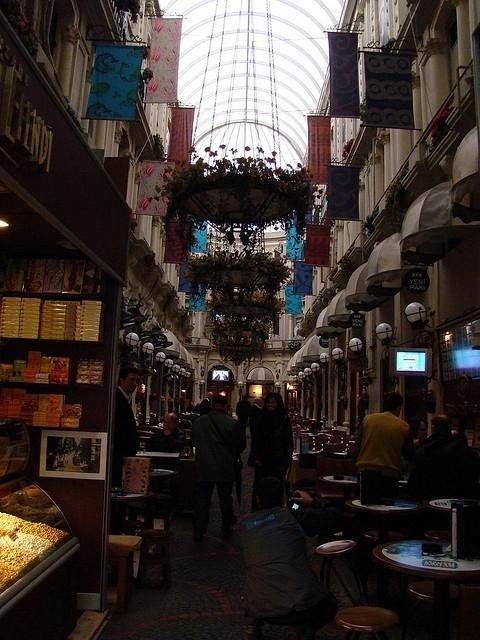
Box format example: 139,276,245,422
374,539,478,639
287,416,360,521
345,495,421,514
427,497,478,513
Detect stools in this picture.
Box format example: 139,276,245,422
363,530,405,549
314,539,369,604
406,580,461,632
102,491,173,613
335,605,402,639
423,530,452,541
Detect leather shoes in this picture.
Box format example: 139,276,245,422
192,515,237,542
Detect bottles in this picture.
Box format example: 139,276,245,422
184,430,194,458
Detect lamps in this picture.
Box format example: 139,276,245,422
119,330,193,379
290,299,434,382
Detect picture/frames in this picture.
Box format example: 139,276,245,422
37,427,109,482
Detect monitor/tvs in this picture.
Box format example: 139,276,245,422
386,348,431,379
210,371,231,382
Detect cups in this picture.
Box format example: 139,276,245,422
139,442,146,452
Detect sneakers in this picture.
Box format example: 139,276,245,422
243,626,314,640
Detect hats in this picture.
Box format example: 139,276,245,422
251,398,264,409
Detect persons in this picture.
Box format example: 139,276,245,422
356,392,412,498
146,412,186,453
199,392,214,416
113,368,141,486
236,395,251,422
411,414,480,499
191,395,246,536
249,392,294,510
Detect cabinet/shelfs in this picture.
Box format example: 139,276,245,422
0,288,109,433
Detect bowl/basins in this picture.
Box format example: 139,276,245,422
111,488,119,498
